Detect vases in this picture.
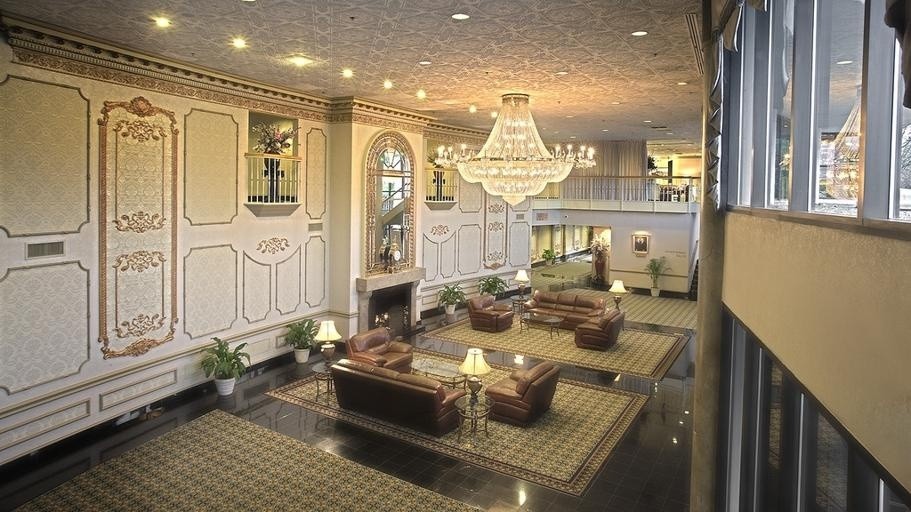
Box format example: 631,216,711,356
264,151,280,170
595,256,605,277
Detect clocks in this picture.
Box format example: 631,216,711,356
378,234,391,263
388,234,402,274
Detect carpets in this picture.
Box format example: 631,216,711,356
260,347,650,497
418,309,692,382
531,261,592,279
551,286,698,331
4,407,486,512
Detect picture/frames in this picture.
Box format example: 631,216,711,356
632,235,648,256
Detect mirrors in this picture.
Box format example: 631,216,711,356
362,127,418,278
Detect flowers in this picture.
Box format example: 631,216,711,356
251,120,301,155
588,233,610,256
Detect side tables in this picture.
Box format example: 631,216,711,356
608,308,628,331
510,294,530,315
454,394,496,445
312,360,335,406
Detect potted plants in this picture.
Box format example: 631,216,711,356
648,155,658,175
424,145,444,178
542,248,556,265
286,319,321,363
436,280,466,315
644,255,671,297
477,275,509,301
199,336,251,396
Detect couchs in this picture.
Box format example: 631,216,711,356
466,294,515,333
575,308,624,351
485,359,561,426
523,289,605,331
331,358,466,438
345,326,413,374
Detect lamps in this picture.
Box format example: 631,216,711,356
313,320,342,370
608,279,629,311
457,348,491,404
826,84,862,200
434,93,596,207
513,269,530,293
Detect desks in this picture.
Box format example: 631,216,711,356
648,182,661,201
262,170,284,202
433,178,446,201
